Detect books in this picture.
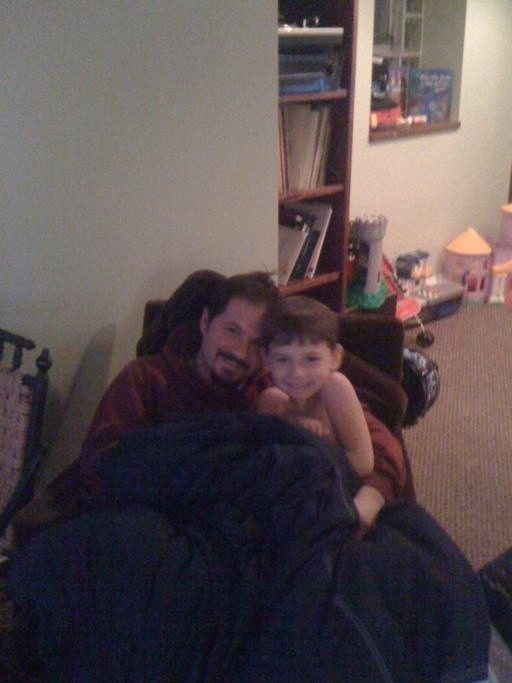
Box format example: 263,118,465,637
279,102,335,196
279,200,333,289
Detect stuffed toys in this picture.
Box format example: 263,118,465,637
396,249,436,294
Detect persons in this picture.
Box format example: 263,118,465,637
254,294,406,543
80,271,280,460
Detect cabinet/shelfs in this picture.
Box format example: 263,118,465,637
278,0,359,317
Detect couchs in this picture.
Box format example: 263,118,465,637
140,300,416,508
